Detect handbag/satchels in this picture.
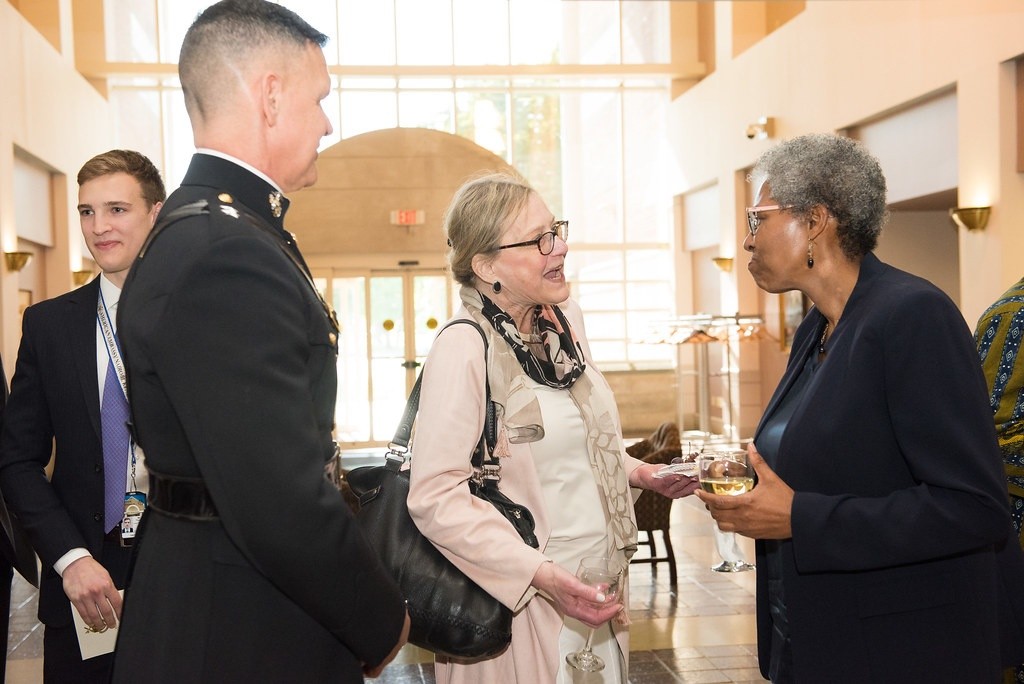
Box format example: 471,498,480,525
340,319,541,664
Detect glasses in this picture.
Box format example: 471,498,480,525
747,201,841,236
492,221,569,256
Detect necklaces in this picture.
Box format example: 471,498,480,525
820,323,828,353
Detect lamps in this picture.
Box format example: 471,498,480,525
949,206,992,232
712,257,733,272
73,270,95,287
5,250,35,272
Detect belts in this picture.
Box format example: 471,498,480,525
146,471,220,521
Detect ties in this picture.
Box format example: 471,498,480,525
103,330,135,532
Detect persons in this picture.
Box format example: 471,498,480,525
693,133,1024,684
123,518,133,533
406,178,700,684
0,150,166,684
973,277,1024,552
113,0,412,684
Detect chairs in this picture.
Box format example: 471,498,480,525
625,422,683,585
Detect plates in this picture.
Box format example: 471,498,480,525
652,464,700,478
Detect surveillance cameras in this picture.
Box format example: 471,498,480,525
746,129,756,138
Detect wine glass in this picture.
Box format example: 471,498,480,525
565,557,624,673
699,450,757,573
